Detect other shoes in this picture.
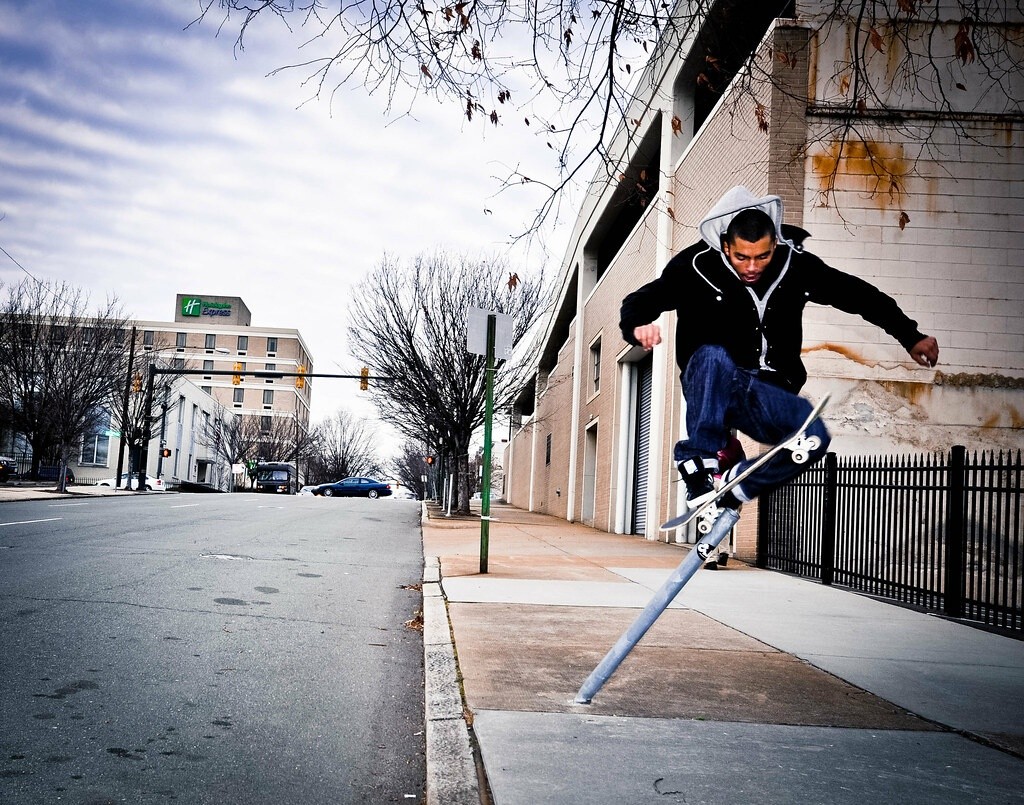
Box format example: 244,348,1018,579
703,561,717,570
717,553,728,567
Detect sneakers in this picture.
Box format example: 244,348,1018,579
672,455,718,509
716,470,743,513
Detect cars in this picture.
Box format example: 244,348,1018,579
94,472,166,491
297,486,317,495
311,477,393,499
490,493,497,499
0,456,19,483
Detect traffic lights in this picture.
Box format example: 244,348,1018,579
159,447,171,458
427,456,435,465
397,480,399,489
132,372,142,392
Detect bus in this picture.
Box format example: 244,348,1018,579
257,462,305,495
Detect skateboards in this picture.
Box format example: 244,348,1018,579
658,391,835,535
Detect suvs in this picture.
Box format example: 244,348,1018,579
21,463,75,484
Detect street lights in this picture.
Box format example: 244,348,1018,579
115,326,229,488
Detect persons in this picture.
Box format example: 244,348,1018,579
619,186,940,508
